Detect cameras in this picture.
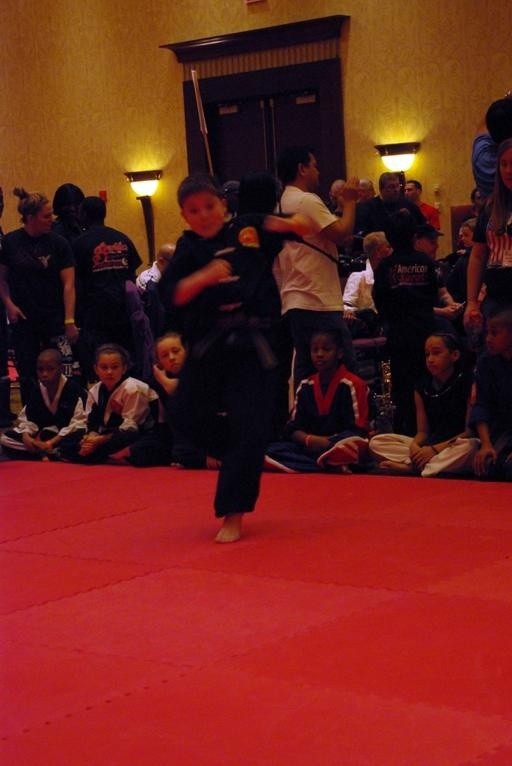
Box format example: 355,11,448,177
339,254,365,276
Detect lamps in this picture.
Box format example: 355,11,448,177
370,139,425,186
121,163,167,269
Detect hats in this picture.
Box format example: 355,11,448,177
414,224,443,240
